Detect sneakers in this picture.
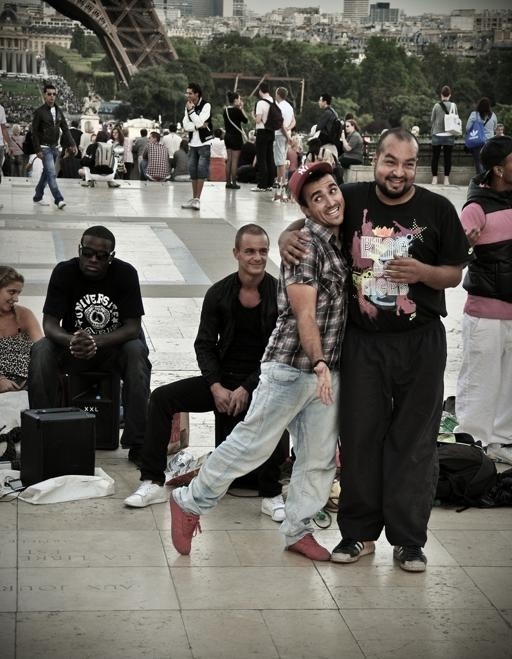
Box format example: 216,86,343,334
393,546,426,571
170,493,201,555
251,187,272,191
487,444,512,465
58,201,65,208
182,198,200,209
38,199,49,206
261,494,375,562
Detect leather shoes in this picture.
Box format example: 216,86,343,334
226,184,240,189
124,480,167,507
107,182,120,187
82,180,94,187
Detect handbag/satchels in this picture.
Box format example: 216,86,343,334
465,111,485,148
242,128,248,144
444,103,463,135
23,132,35,154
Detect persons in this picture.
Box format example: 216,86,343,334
125,222,286,523
1,265,46,436
279,125,470,572
27,225,152,464
171,161,349,562
459,135,512,468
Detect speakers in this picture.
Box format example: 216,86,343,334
20,406,97,487
59,369,121,452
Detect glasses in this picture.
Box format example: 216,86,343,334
81,244,114,260
47,93,56,95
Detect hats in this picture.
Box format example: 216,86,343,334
480,136,512,168
289,162,332,201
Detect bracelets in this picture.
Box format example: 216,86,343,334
314,359,332,371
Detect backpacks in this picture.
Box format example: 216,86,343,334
325,107,342,142
434,433,496,512
255,97,283,130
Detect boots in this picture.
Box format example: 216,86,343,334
444,176,449,185
432,176,437,184
